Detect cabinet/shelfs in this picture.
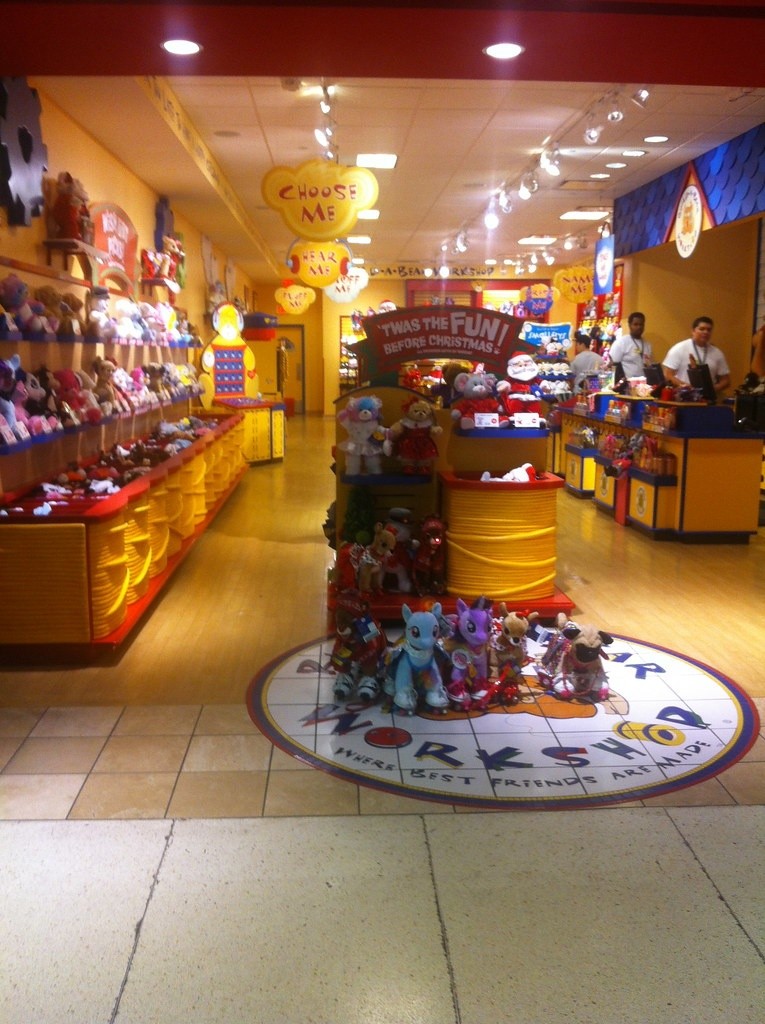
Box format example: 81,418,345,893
335,385,575,620
0,237,206,456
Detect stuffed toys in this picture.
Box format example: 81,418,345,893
140,233,186,280
0,273,219,515
52,170,97,245
334,350,615,714
530,341,572,399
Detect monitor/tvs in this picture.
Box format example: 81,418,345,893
688,363,718,401
610,362,627,391
643,362,666,386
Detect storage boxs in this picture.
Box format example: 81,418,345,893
437,468,565,600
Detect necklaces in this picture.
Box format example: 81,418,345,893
631,336,644,365
693,341,708,364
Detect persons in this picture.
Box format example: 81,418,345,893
569,335,606,374
608,312,651,381
661,317,731,397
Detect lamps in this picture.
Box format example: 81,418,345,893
314,84,655,279
